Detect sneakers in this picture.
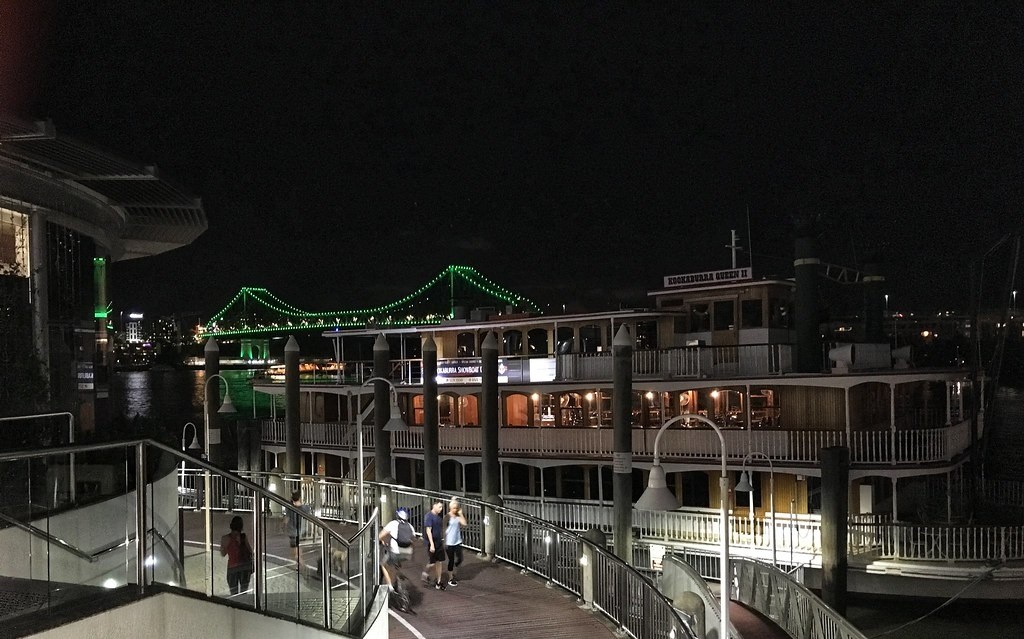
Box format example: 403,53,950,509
421,571,459,591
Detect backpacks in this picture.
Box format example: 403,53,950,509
389,519,413,547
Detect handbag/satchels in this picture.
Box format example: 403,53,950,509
237,533,253,571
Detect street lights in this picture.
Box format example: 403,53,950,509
181,422,202,506
733,452,776,567
633,414,733,639
203,374,237,467
355,377,409,532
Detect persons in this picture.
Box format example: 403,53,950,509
420,499,447,591
377,508,417,595
284,491,311,565
219,515,254,596
442,500,467,586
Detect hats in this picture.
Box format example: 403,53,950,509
291,490,300,501
